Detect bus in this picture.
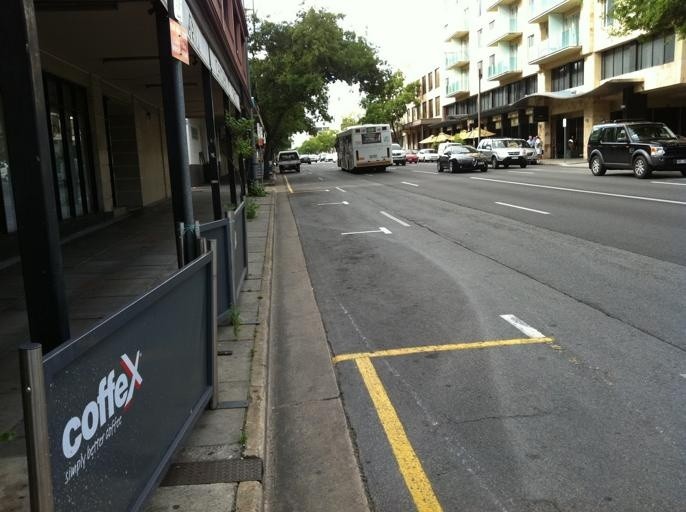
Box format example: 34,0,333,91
333,123,393,173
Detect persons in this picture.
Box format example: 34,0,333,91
616,126,638,141
526,135,543,164
567,135,576,158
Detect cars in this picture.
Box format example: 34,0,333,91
392,138,538,173
276,150,337,174
587,118,686,179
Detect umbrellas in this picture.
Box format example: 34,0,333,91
418,127,496,144
